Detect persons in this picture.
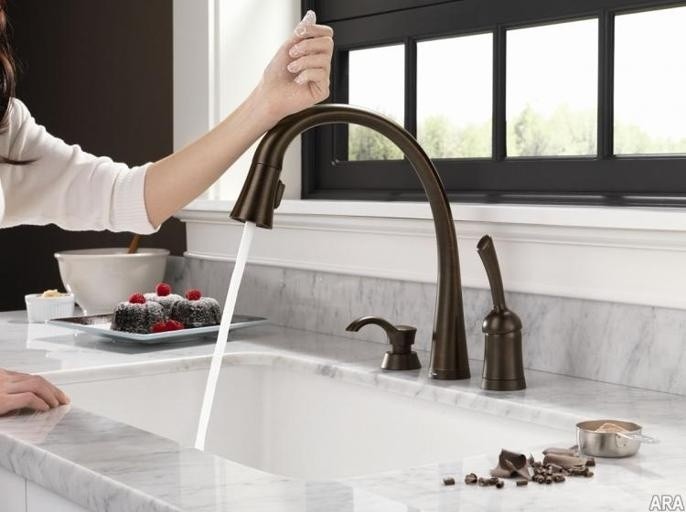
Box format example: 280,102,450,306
2,0,334,417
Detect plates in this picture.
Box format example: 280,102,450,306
45,312,267,345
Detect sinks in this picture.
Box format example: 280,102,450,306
36,352,591,484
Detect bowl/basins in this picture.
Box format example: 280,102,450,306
575,420,659,459
53,246,171,317
22,293,74,323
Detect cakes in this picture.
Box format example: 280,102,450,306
110,282,223,334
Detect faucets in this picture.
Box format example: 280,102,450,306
227,102,474,382
